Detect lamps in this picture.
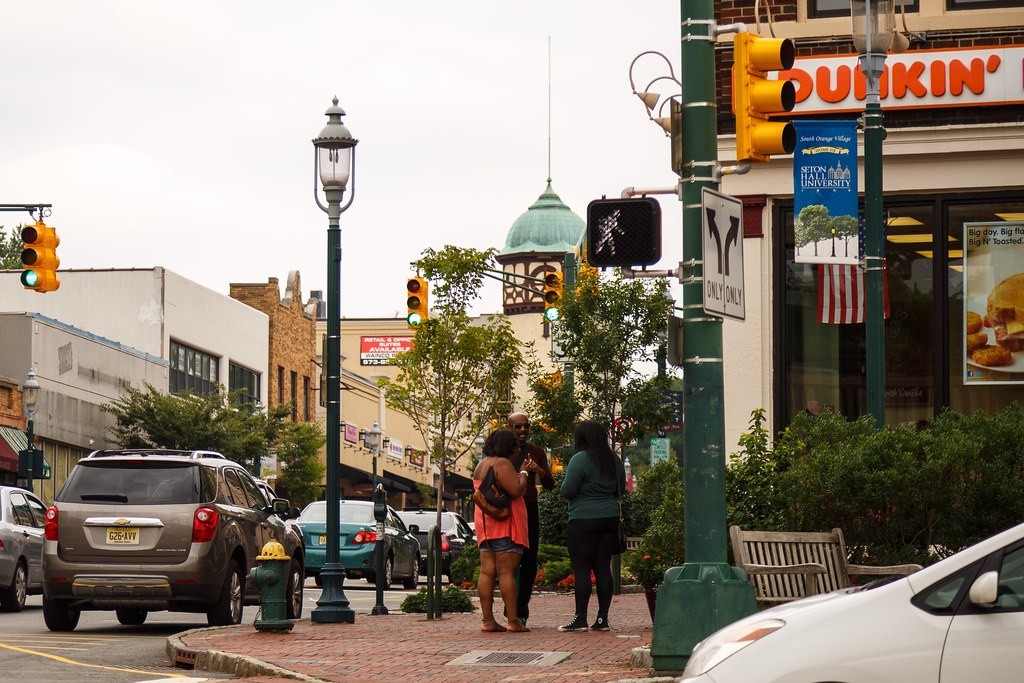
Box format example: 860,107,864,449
887,0,926,52
359,429,366,440
340,420,346,433
405,445,411,457
383,436,390,447
430,455,435,464
629,51,683,137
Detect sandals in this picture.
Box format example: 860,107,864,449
481,618,508,631
507,618,530,631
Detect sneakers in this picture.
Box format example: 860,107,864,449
590,613,610,631
557,613,589,631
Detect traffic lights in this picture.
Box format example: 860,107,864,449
35,226,60,293
585,196,662,267
730,30,799,164
20,224,47,291
406,277,429,330
544,271,565,324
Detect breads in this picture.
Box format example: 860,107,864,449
967,311,1012,367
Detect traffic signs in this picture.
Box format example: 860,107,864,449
701,186,747,325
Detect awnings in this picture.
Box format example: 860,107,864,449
0,424,51,479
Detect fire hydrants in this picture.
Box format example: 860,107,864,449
244,535,297,634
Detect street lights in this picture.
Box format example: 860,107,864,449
309,94,362,624
367,420,383,489
21,367,42,493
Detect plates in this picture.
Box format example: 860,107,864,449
967,294,1024,373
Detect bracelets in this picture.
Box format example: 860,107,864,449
519,470,528,477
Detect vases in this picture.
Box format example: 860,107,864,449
644,583,657,624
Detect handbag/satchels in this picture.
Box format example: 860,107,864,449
611,521,628,555
473,456,511,521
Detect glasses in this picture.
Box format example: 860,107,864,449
509,423,532,429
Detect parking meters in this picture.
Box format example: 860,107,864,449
371,488,389,615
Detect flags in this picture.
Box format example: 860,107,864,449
817,207,891,324
795,121,859,264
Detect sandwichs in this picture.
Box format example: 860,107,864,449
983,272,1024,352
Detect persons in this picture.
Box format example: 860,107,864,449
501,411,556,626
558,420,626,631
473,428,530,632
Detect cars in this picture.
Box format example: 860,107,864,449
0,486,49,612
395,507,478,586
292,499,423,590
679,520,1024,683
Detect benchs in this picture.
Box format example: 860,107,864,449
730,526,923,612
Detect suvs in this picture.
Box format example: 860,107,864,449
39,448,306,633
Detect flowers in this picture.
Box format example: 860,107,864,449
626,519,686,586
